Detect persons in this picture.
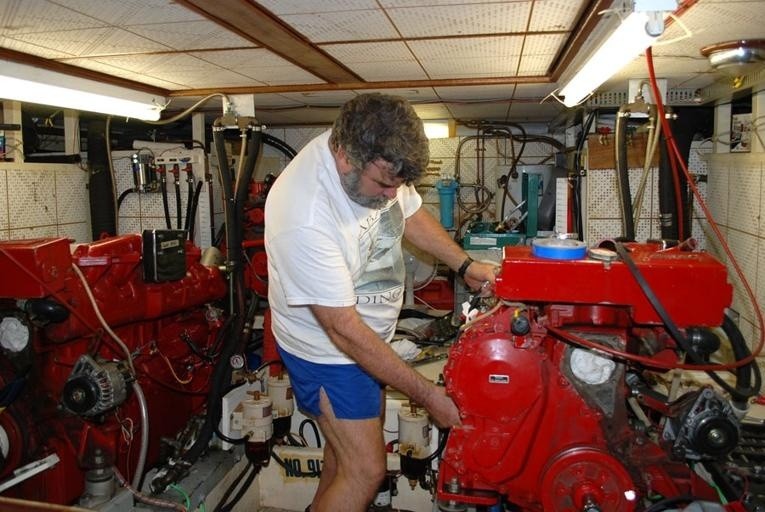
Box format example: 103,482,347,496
262,89,503,511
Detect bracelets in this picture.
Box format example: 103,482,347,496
455,254,475,279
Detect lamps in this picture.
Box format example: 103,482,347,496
700,41,764,77
